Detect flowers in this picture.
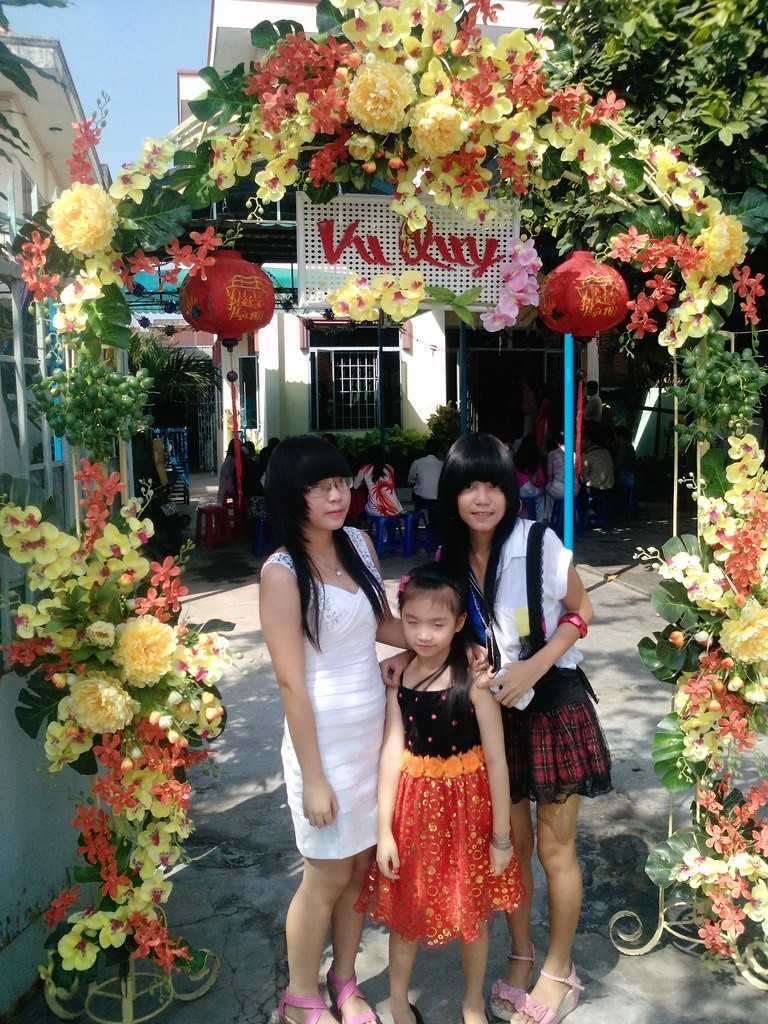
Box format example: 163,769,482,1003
631,434,768,996
0,460,236,1024
0,0,768,460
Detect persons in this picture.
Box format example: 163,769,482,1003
217,380,637,533
352,560,529,1024
378,434,613,1024
259,434,490,1024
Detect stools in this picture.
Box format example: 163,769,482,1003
393,511,434,557
414,505,440,544
195,493,249,550
516,486,651,538
251,516,274,560
369,517,407,561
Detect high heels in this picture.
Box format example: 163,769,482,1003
509,958,586,1024
489,941,535,1021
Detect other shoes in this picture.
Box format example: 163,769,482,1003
409,1003,424,1024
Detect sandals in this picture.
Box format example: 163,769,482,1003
326,961,378,1023
278,986,329,1024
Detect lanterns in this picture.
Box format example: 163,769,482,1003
179,250,274,506
537,252,629,487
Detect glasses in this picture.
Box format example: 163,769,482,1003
307,476,354,498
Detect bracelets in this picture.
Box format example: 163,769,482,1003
491,831,513,850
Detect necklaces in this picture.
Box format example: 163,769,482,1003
470,582,494,668
307,550,342,576
